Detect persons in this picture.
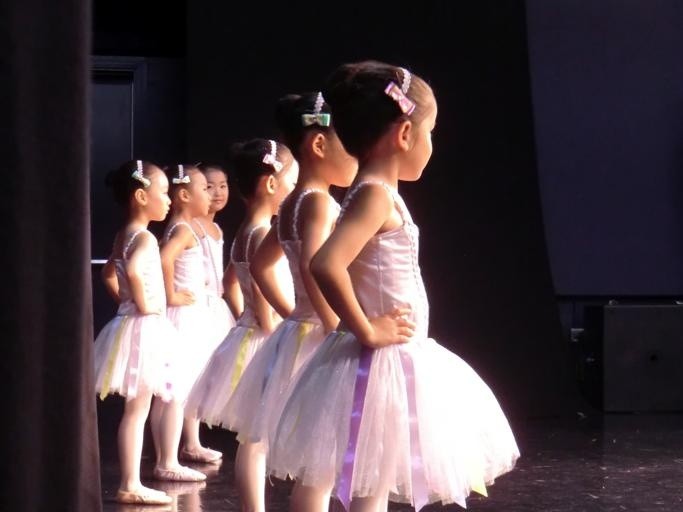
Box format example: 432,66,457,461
178,160,238,462
92,157,179,504
266,60,523,512
217,88,360,512
182,136,300,512
153,162,222,481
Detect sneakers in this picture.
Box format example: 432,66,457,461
116,485,173,505
152,465,207,482
180,447,223,463
